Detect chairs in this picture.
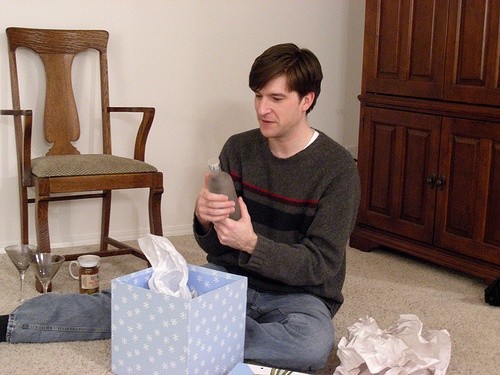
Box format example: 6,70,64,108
0,27,165,292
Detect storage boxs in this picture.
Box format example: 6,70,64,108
111,263,248,375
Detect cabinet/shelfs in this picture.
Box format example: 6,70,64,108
349,0,500,284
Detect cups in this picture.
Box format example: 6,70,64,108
69,255,101,279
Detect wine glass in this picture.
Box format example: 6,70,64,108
28,253,65,294
5,244,40,301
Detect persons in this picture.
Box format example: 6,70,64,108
2,42,362,372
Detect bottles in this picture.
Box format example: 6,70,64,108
206,159,241,221
79,264,99,295
